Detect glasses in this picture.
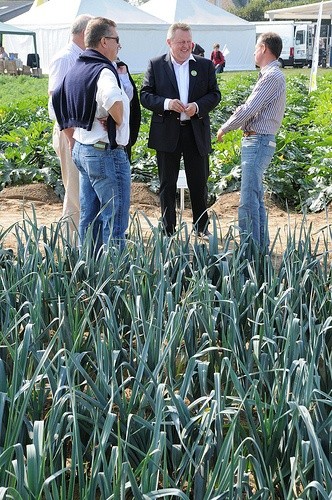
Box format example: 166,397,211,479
99,36,119,43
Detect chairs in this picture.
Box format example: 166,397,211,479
0,60,23,77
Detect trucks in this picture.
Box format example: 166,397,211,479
247,20,318,68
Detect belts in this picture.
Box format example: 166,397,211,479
244,132,256,136
179,121,192,125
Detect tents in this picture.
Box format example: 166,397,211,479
0,21,39,69
2,0,173,75
137,0,256,70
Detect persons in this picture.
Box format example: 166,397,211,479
217,32,286,256
48,14,134,245
192,42,205,57
210,44,224,73
0,46,8,60
139,23,221,241
52,17,131,260
